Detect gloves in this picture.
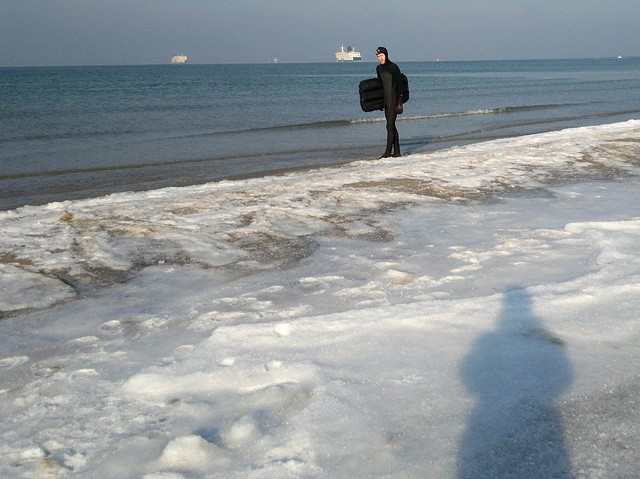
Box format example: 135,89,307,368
395,93,403,114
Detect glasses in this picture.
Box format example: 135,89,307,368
376,50,386,55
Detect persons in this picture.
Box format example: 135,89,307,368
375,46,405,160
